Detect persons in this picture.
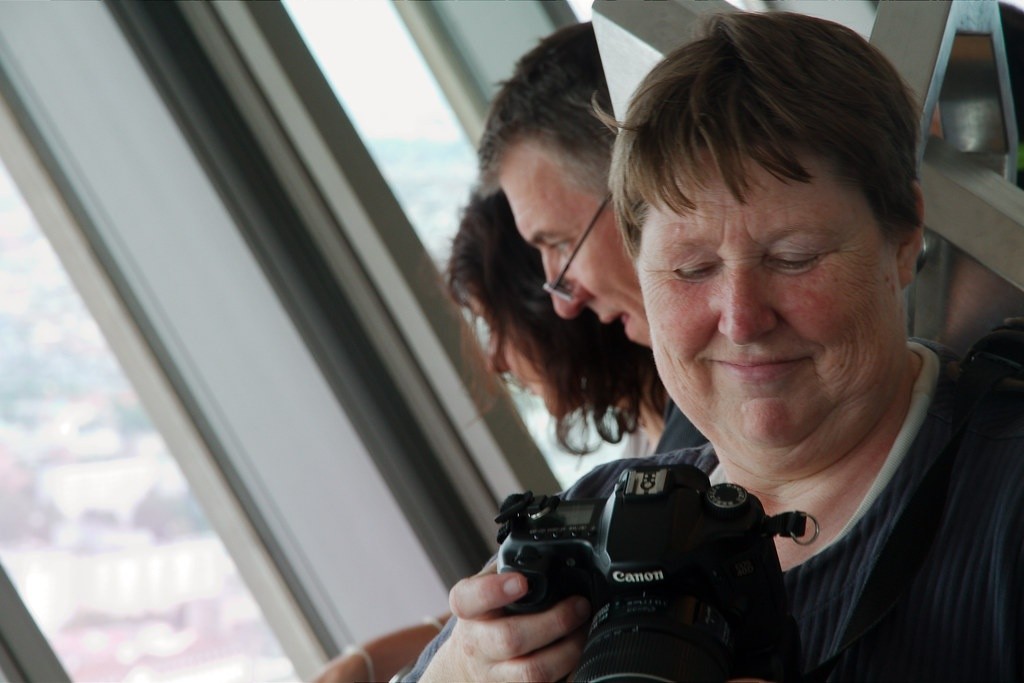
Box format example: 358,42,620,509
477,21,712,454
314,179,709,682
385,12,1024,683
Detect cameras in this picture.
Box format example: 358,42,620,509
496,462,806,683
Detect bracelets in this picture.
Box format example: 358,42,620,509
419,617,443,632
346,646,375,682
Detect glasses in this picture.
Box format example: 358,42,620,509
542,192,613,301
